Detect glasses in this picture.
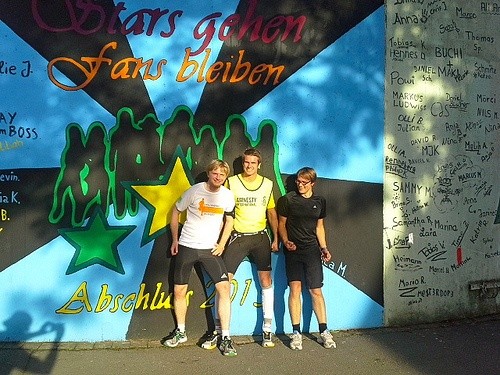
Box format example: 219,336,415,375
294,177,311,186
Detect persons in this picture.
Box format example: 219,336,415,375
200,148,281,350
162,159,238,357
275,167,337,350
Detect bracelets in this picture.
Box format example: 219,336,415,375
321,247,329,252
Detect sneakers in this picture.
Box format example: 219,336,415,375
219,338,237,357
263,329,275,347
203,331,223,350
163,329,187,348
289,330,303,350
317,330,337,350
